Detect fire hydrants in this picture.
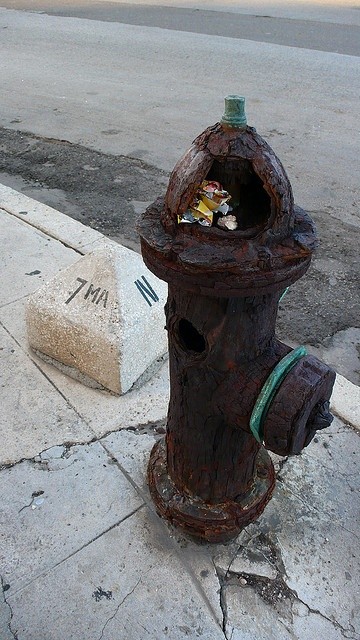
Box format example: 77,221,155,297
137,95,336,545
